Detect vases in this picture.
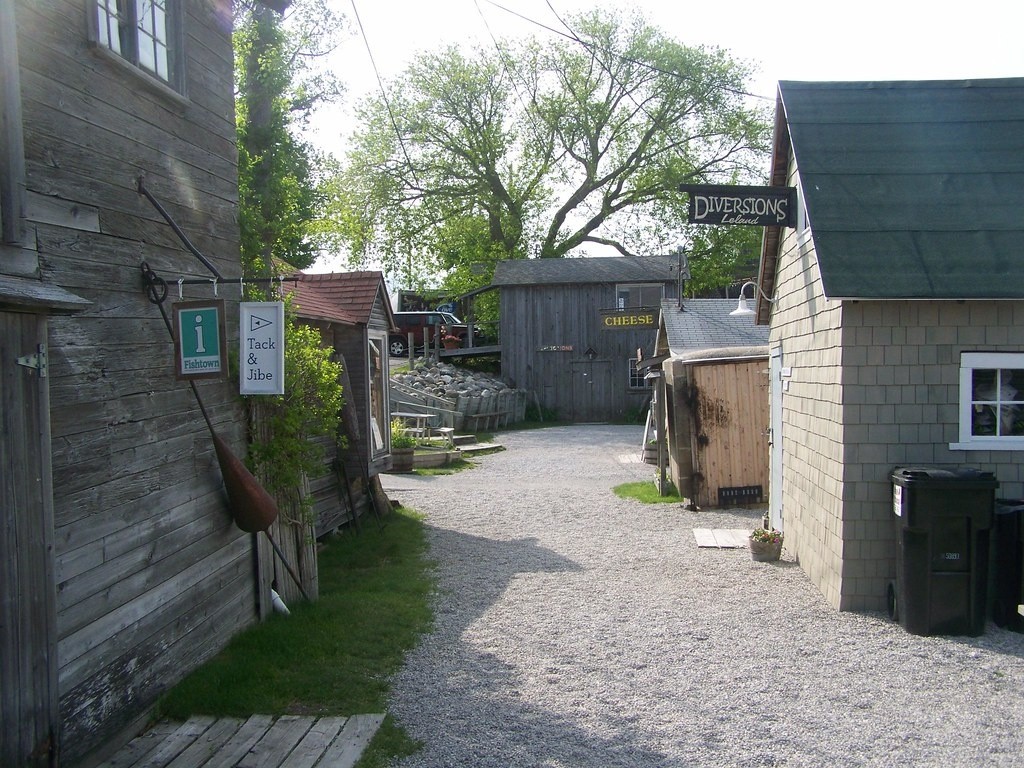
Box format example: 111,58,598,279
748,536,782,561
445,343,456,350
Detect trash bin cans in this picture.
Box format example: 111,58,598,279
991,494,1024,634
886,461,1001,640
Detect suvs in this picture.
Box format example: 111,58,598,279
388,312,479,357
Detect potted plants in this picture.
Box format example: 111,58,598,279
384,418,415,474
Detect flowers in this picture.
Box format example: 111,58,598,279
752,528,783,544
441,335,462,342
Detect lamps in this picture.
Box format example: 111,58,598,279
727,282,779,316
